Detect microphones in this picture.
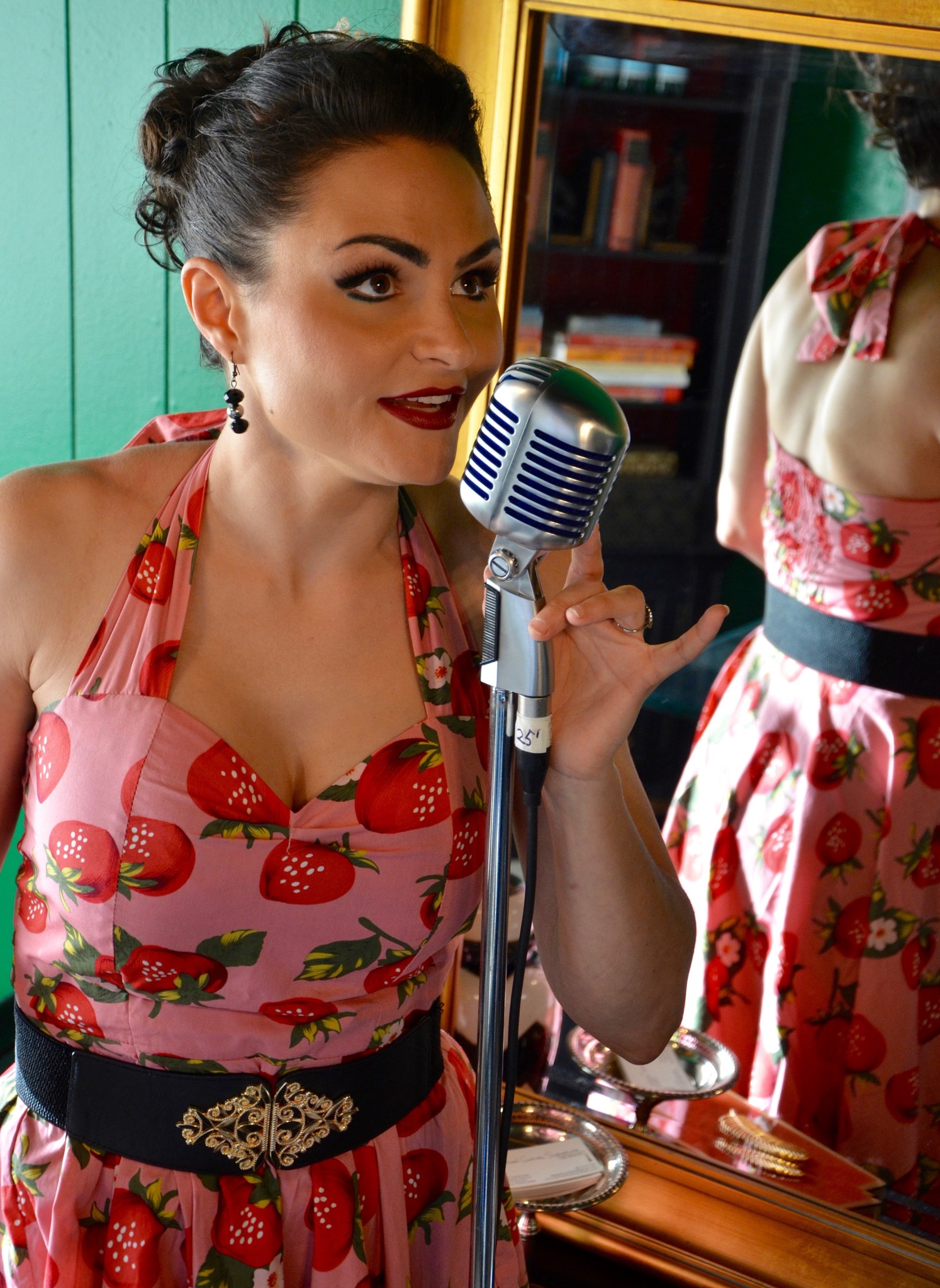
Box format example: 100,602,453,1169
452,364,632,592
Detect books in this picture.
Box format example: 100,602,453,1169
515,304,698,406
576,120,657,254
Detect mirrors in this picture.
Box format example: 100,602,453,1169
396,0,940,1288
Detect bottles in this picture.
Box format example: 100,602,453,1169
550,54,691,252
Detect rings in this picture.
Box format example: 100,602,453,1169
614,604,653,634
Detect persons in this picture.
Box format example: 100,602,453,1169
663,52,940,1244
0,19,728,1288
